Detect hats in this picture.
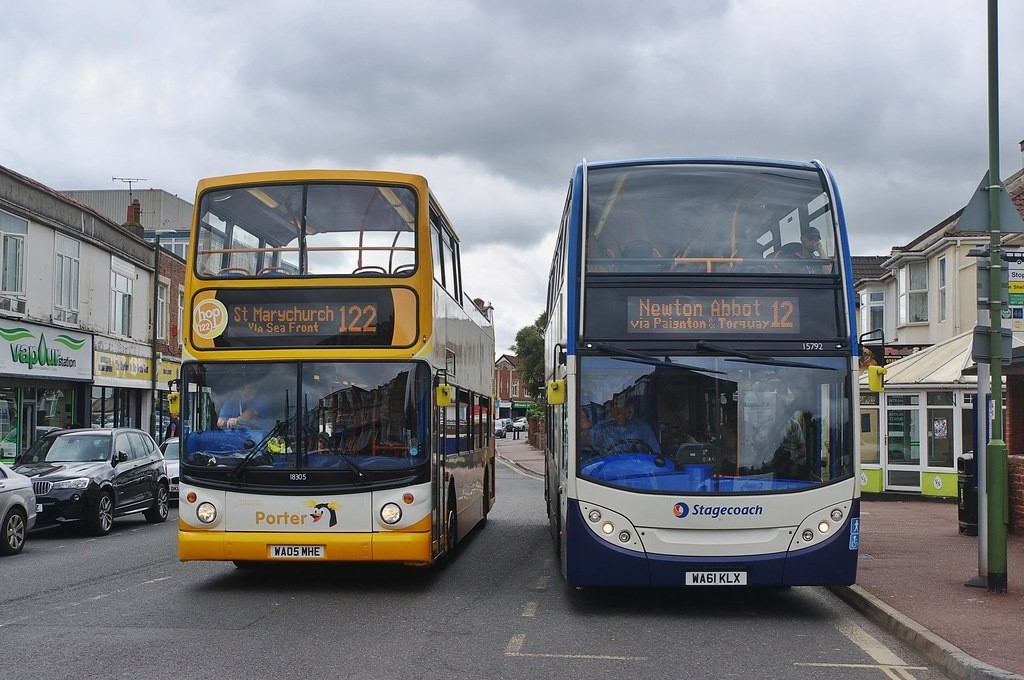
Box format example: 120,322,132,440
803,227,822,240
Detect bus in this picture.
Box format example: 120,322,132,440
168,169,497,567
539,158,887,588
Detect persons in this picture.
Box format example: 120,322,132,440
475,298,494,312
165,413,179,440
217,379,274,431
318,432,333,455
590,393,661,454
581,376,606,446
754,404,806,479
779,227,832,274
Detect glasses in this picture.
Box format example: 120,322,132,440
805,235,820,241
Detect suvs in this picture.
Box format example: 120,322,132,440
11,429,169,536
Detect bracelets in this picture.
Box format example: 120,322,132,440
795,455,798,458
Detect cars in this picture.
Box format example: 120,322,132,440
0,462,37,555
92,411,170,437
495,417,529,438
0,426,63,457
159,437,179,501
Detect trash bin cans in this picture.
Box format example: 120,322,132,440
957,452,978,536
